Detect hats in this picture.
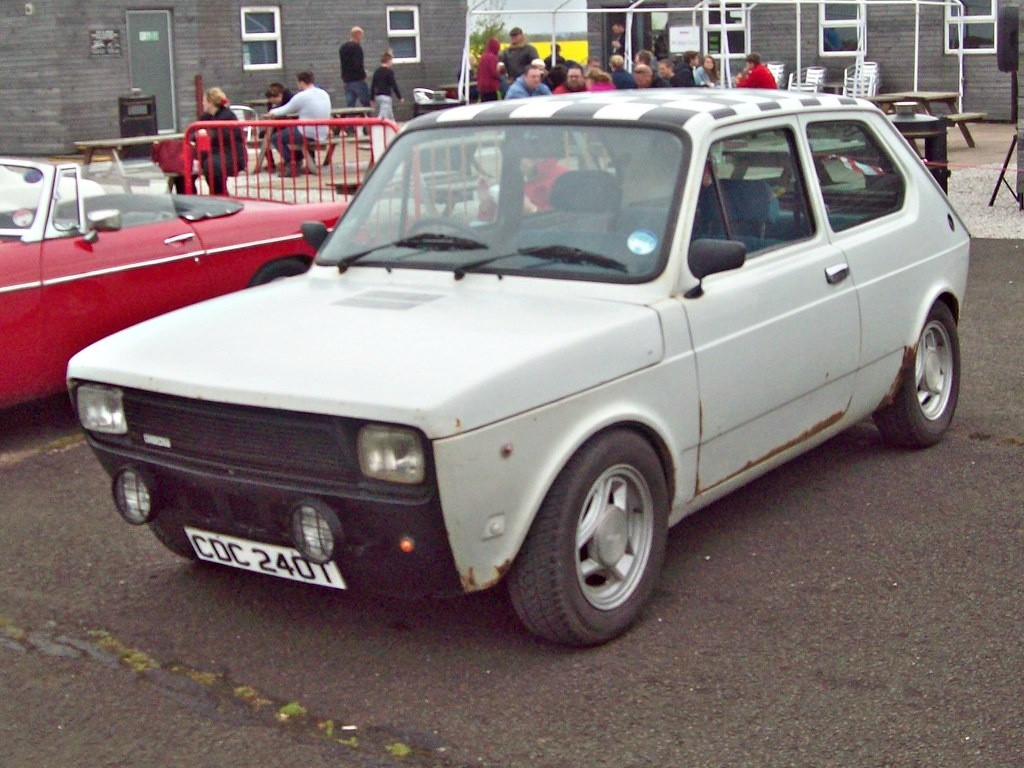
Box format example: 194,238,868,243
531,59,545,67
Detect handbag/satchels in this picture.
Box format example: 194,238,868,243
152,139,194,174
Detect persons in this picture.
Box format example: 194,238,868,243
475,36,512,102
173,83,248,196
258,70,332,177
543,59,589,95
504,58,555,99
500,27,540,81
733,52,778,89
692,55,719,87
669,50,715,88
653,57,687,88
467,52,476,102
630,49,667,88
610,21,639,62
544,44,567,71
645,28,668,58
584,67,617,91
608,54,638,89
338,27,377,135
585,56,603,69
370,50,405,130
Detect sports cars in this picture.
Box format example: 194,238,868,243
0,155,350,409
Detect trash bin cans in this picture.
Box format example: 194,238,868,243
118,93,157,158
411,89,474,203
865,101,947,193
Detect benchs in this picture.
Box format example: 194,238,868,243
246,134,504,211
929,111,987,124
64,162,200,181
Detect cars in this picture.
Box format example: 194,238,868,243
63,87,971,650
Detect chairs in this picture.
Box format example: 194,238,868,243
510,170,624,252
697,179,782,249
230,103,259,140
766,61,885,98
412,85,446,102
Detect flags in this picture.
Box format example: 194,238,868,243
830,152,887,176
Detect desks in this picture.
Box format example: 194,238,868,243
823,81,845,94
262,102,377,177
244,97,271,118
412,98,467,120
74,133,187,196
439,82,477,99
866,91,977,150
